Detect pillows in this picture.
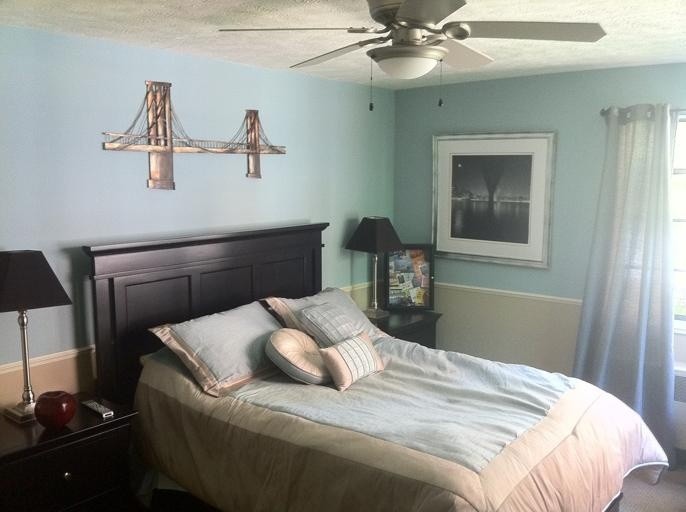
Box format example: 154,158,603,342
146,300,284,397
259,287,390,347
318,330,384,392
300,301,359,347
265,327,333,386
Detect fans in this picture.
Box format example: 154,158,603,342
218,1,607,72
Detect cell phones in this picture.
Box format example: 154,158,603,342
81,398,115,420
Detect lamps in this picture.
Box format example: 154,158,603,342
0,250,73,425
367,44,449,112
344,216,401,321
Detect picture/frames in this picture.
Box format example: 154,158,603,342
385,244,434,313
431,130,559,270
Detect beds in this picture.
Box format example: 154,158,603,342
82,221,669,512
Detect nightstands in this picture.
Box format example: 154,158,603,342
0,392,139,512
364,308,442,350
